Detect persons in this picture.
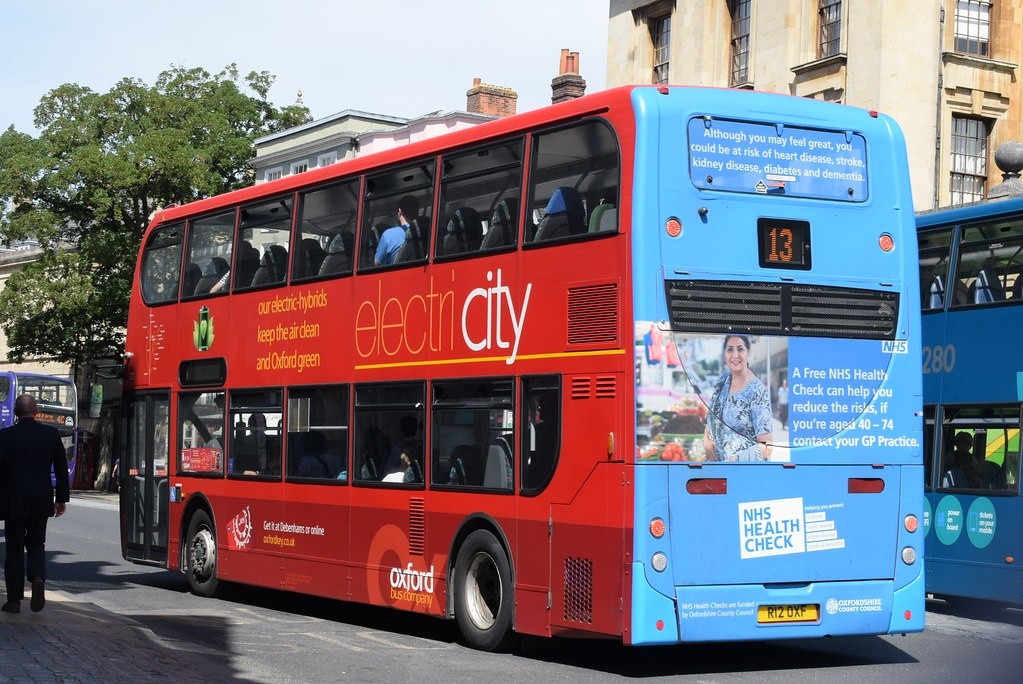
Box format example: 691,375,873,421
0,393,71,612
112,459,121,493
240,409,552,494
704,335,773,464
373,195,422,267
210,241,251,293
777,380,790,431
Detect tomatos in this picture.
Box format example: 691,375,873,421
661,442,687,463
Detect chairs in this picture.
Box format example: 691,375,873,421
923,266,1023,310
293,426,515,490
34,396,62,406
973,460,1004,487
166,186,617,300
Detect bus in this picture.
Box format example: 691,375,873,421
88,84,926,652
910,194,1023,616
0,370,77,490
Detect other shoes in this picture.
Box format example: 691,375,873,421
30,576,46,613
2,601,21,614
